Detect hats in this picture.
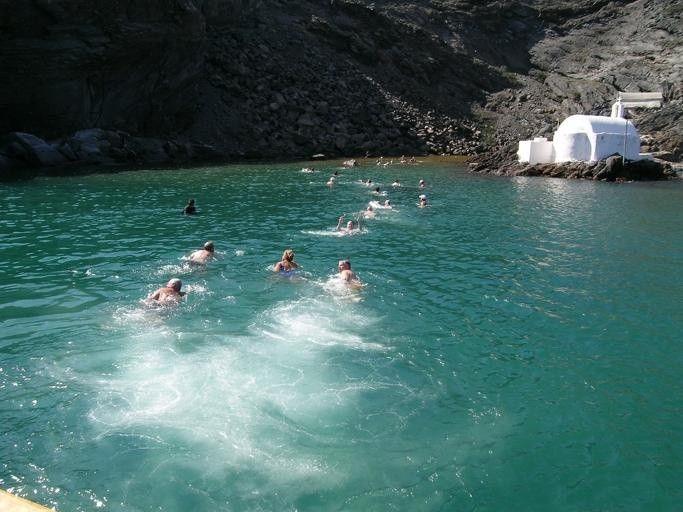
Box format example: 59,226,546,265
418,195,427,199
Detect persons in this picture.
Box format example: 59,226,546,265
301,154,429,234
337,259,361,287
272,248,300,278
180,198,196,215
144,276,183,305
184,240,215,264
